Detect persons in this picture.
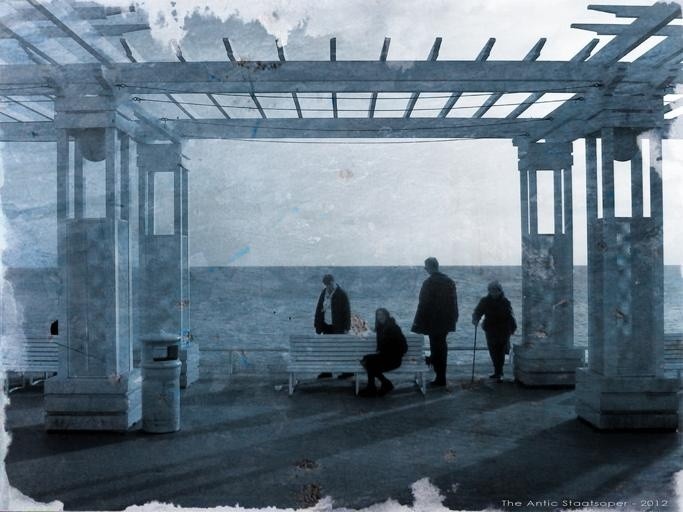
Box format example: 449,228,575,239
410,256,458,389
313,275,356,380
471,281,517,380
357,308,409,395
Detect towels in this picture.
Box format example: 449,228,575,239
137,332,182,434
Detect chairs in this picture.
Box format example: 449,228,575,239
490,367,504,384
318,371,333,379
337,372,355,380
360,380,394,397
426,379,447,391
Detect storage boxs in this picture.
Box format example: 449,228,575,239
285,334,431,395
